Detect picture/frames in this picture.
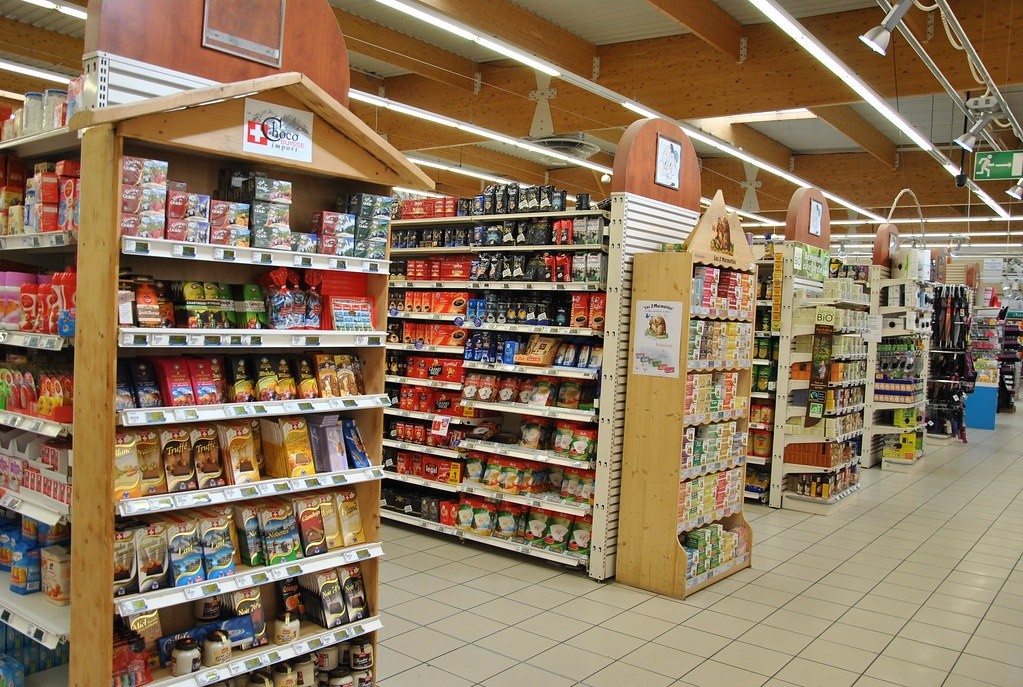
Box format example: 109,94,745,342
888,232,897,260
808,198,823,237
655,131,682,192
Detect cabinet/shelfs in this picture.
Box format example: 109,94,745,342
379,191,700,581
1,73,434,687
925,281,1023,439
745,240,872,516
853,265,934,469
616,190,759,602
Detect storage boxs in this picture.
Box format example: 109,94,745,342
2,156,391,686
383,179,930,585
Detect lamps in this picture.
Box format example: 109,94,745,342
1005,177,1023,200
858,0,914,57
401,151,599,207
0,58,71,86
349,88,773,223
954,112,1005,153
374,0,883,220
21,1,89,22
748,0,1010,220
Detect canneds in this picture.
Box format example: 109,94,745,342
170,612,373,687
22,89,68,134
